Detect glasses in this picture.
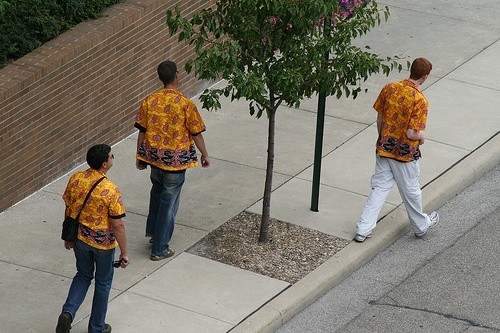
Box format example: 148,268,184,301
112,258,127,268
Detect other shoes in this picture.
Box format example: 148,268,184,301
56,312,73,333
99,324,112,333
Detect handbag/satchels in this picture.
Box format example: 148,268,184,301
60,216,78,242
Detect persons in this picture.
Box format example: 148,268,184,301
134,60,209,261
354,57,439,243
55,143,130,333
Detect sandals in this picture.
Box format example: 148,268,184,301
150,248,174,260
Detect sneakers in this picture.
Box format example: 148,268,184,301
354,230,372,242
414,211,439,238
